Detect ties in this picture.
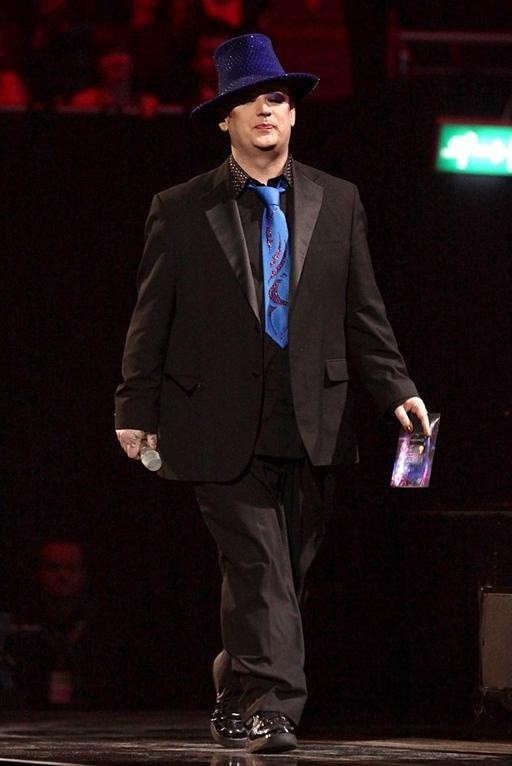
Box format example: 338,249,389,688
246,179,292,349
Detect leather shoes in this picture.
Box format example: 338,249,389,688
247,711,297,754
210,651,249,748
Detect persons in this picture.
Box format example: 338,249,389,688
0,0,246,120
2,536,108,715
114,35,431,755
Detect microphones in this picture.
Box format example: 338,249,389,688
133,438,164,475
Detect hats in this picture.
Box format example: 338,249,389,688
191,33,321,122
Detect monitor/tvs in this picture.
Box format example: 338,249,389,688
432,116,511,179
